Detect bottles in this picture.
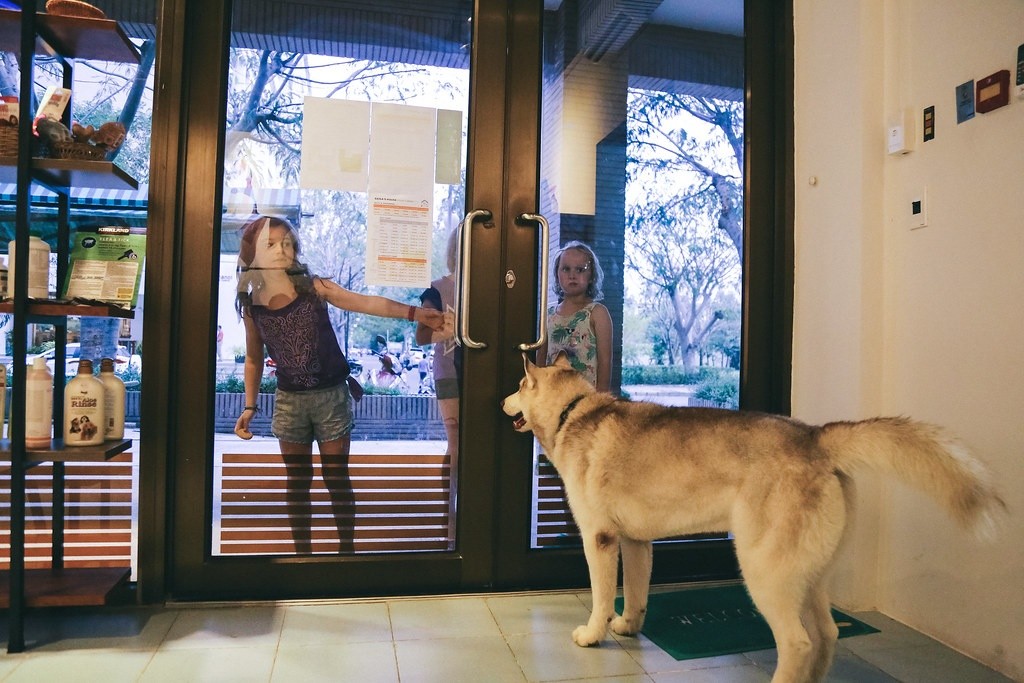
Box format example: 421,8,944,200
24,357,52,448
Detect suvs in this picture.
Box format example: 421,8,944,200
8,341,142,378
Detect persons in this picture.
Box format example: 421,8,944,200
415,224,462,551
217,325,223,362
535,240,614,397
360,351,362,357
234,216,455,554
418,354,430,393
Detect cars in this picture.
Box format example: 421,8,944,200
232,349,279,382
404,347,437,369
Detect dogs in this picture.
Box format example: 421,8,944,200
498,350,1011,683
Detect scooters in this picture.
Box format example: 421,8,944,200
347,335,414,394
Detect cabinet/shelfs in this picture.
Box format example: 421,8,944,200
0,0,138,654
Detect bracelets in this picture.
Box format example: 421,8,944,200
408,305,416,322
241,405,258,413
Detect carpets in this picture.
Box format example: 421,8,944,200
614,584,881,662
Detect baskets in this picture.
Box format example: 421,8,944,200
46,0,106,19
55,141,105,160
0,124,18,156
